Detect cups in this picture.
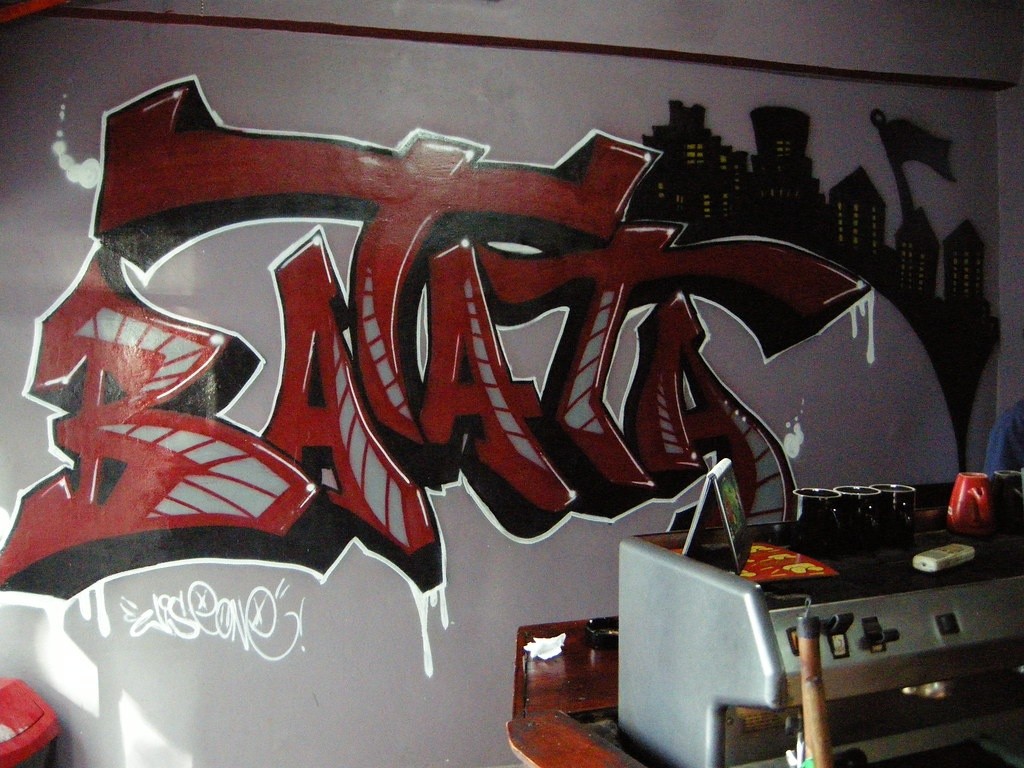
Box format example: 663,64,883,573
946,472,997,535
832,486,882,551
992,469,1024,531
790,487,843,554
869,483,915,546
